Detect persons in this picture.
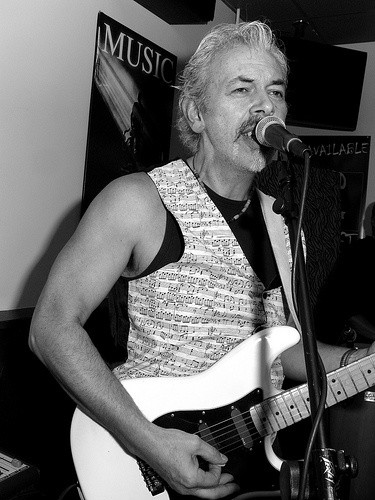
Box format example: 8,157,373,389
27,20,375,499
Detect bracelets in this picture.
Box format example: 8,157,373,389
340,347,357,368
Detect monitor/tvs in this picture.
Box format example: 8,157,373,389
283,37,367,133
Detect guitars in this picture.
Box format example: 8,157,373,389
69,325,375,500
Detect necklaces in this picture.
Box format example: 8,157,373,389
193,151,256,224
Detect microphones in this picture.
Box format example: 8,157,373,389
252,115,312,159
